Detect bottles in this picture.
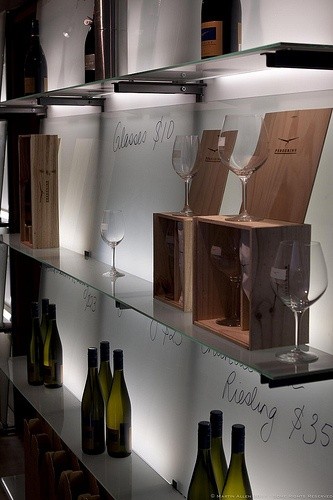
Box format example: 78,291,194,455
98,341,112,412
222,424,253,500
201,0,242,59
209,410,229,500
84,11,95,84
27,302,43,386
106,349,131,459
80,347,104,456
40,298,50,344
19,20,45,97
42,304,62,389
187,421,219,500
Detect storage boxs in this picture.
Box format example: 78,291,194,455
192,216,311,350
153,213,192,312
18,135,58,249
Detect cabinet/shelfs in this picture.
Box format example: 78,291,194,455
0,42,333,500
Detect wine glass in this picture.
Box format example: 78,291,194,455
210,227,243,327
99,208,125,277
269,240,328,363
172,135,207,217
164,221,174,300
217,113,270,223
